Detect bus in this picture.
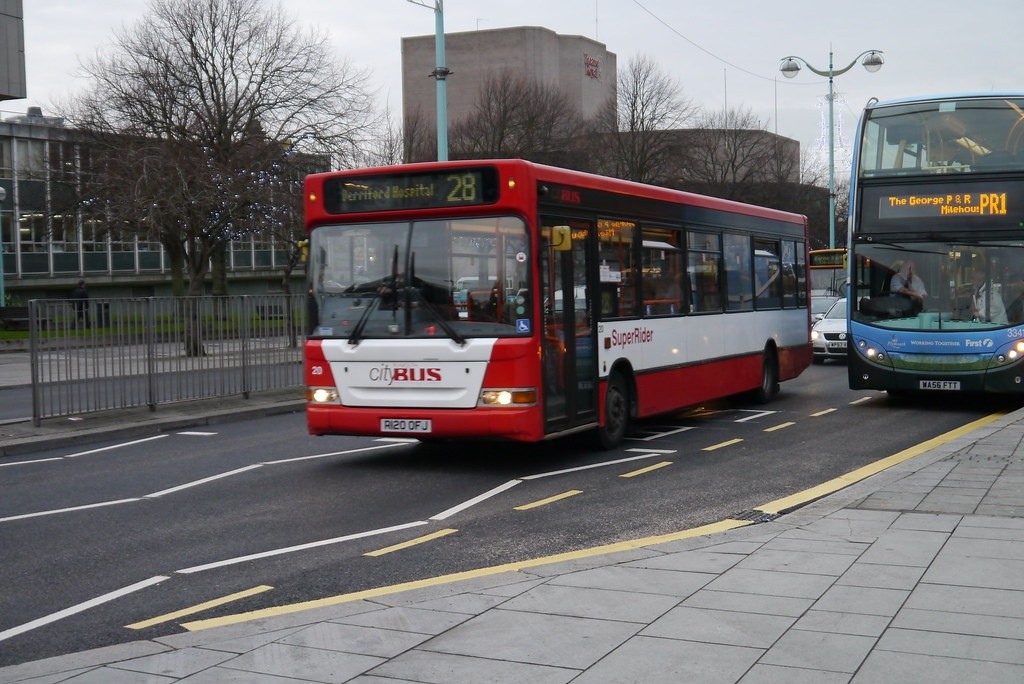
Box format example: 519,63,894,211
303,159,814,450
846,88,1024,403
808,251,983,303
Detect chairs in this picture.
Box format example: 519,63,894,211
647,277,688,315
697,273,723,311
976,150,1013,172
727,271,741,309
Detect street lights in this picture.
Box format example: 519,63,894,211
779,42,884,249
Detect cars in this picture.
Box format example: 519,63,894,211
811,297,871,365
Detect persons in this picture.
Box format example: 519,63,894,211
890,260,928,310
969,270,1009,326
